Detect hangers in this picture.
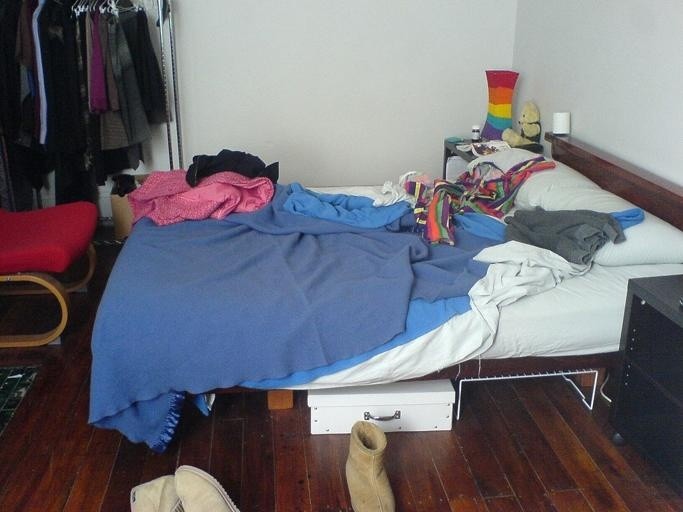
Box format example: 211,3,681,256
73,1,158,17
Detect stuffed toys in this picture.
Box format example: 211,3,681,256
502,102,541,146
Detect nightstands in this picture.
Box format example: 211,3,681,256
607,275,683,499
443,138,544,180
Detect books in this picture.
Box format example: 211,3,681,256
456,141,511,157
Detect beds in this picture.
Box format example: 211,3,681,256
88,132,683,457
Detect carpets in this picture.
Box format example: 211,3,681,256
0,364,42,437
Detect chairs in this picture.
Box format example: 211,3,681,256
0,202,98,347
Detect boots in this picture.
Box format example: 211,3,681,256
345,420,396,511
129,465,239,511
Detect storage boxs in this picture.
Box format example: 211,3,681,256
307,379,456,435
111,175,149,241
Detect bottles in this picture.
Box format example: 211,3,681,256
472,126,480,141
